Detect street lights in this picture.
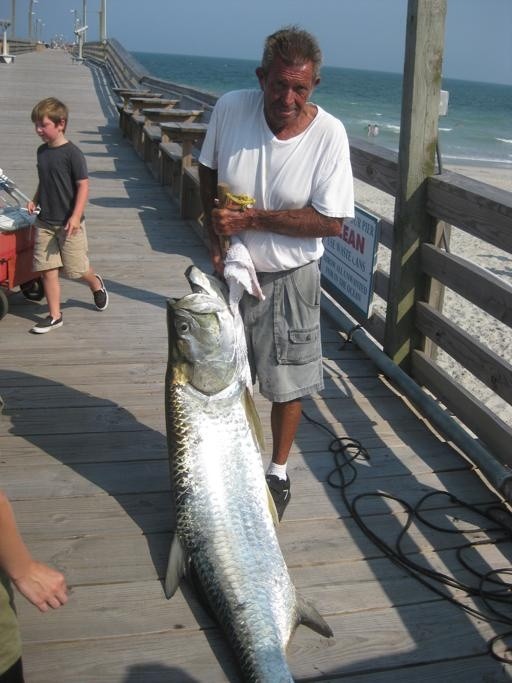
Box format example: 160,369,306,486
71,9,81,44
31,0,46,42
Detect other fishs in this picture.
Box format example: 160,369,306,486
164,264,334,683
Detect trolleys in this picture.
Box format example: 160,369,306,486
0,170,47,319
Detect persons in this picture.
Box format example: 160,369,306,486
0,489,70,683
196,28,356,527
368,124,379,137
24,96,108,334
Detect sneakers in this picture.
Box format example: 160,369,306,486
93,274,109,311
265,475,291,521
32,312,64,334
